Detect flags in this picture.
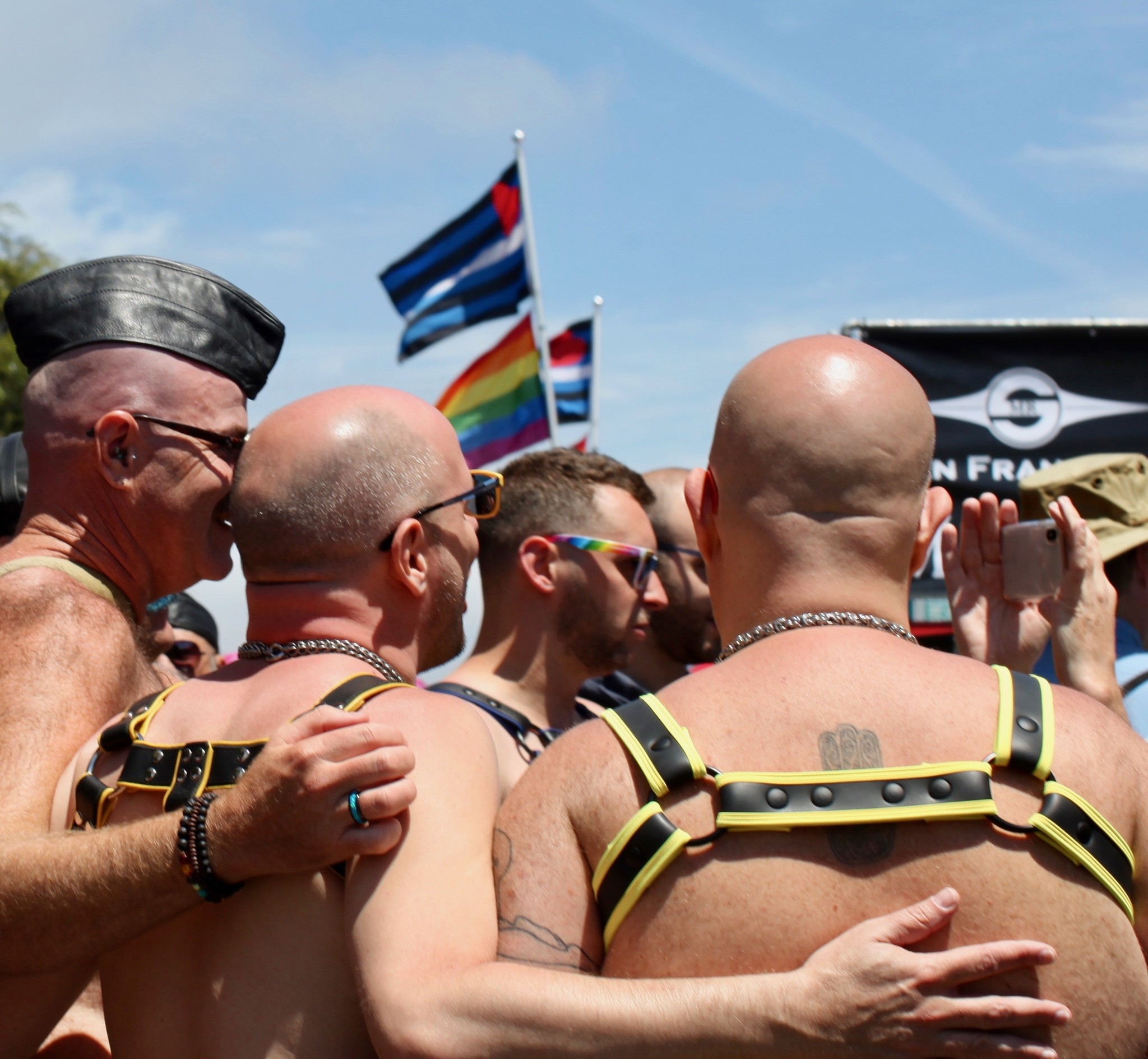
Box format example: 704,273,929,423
549,317,592,454
436,309,553,470
376,160,537,364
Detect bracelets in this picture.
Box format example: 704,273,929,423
177,790,246,904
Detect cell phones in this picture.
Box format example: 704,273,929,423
1001,519,1068,600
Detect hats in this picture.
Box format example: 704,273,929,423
1017,452,1148,563
3,254,286,401
0,431,30,505
167,592,218,653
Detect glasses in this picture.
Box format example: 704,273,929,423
86,414,254,466
379,470,504,552
165,641,218,676
544,534,659,590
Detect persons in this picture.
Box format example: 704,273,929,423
0,256,418,1059
48,253,1148,1059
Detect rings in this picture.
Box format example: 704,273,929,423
348,792,369,828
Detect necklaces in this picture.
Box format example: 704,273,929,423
239,638,407,683
715,610,921,665
